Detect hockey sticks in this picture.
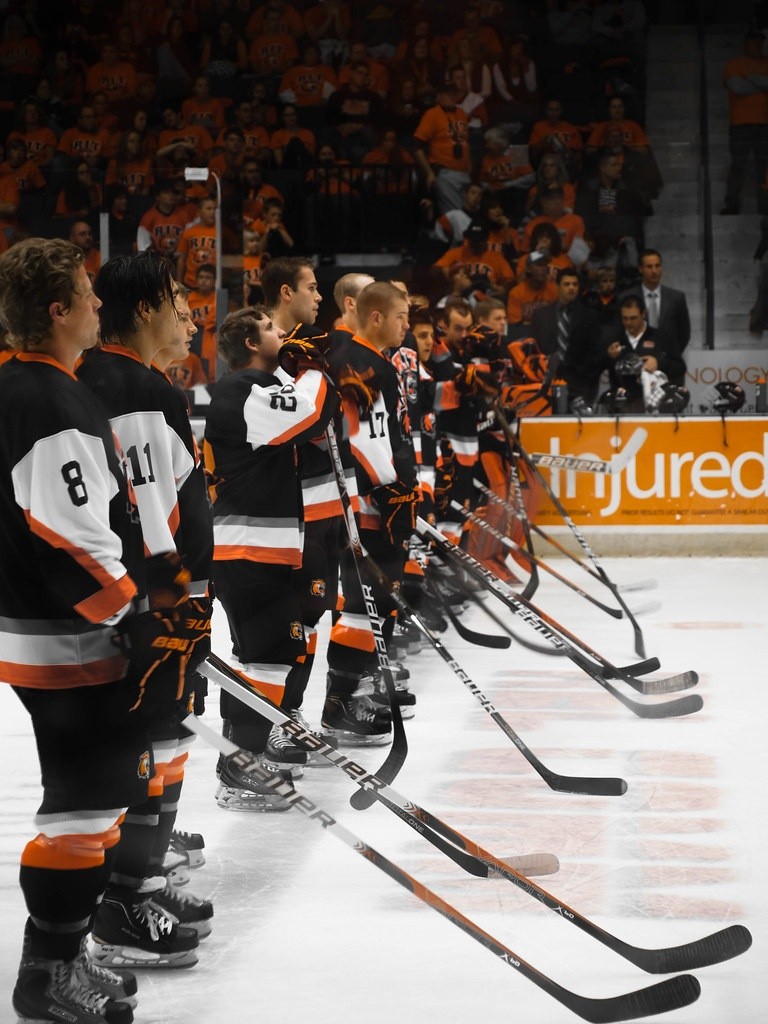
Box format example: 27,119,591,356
323,397,704,811
195,650,752,975
167,703,701,1024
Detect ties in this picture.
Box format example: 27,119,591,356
553,307,570,365
648,295,659,328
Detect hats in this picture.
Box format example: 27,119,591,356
525,250,549,264
462,217,488,235
744,29,766,40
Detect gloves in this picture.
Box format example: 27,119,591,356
278,323,334,373
465,261,495,289
463,322,501,360
371,481,437,552
116,599,209,729
338,362,373,411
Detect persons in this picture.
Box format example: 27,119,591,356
1,238,150,1024
719,31,768,215
149,272,206,885
208,259,541,813
0,0,696,413
76,244,213,974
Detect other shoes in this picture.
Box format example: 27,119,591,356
720,204,740,215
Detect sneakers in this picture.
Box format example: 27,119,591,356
388,561,488,658
374,664,410,691
368,666,415,718
11,672,392,1023
351,669,392,721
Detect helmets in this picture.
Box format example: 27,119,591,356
598,387,634,414
712,382,746,412
657,382,690,413
